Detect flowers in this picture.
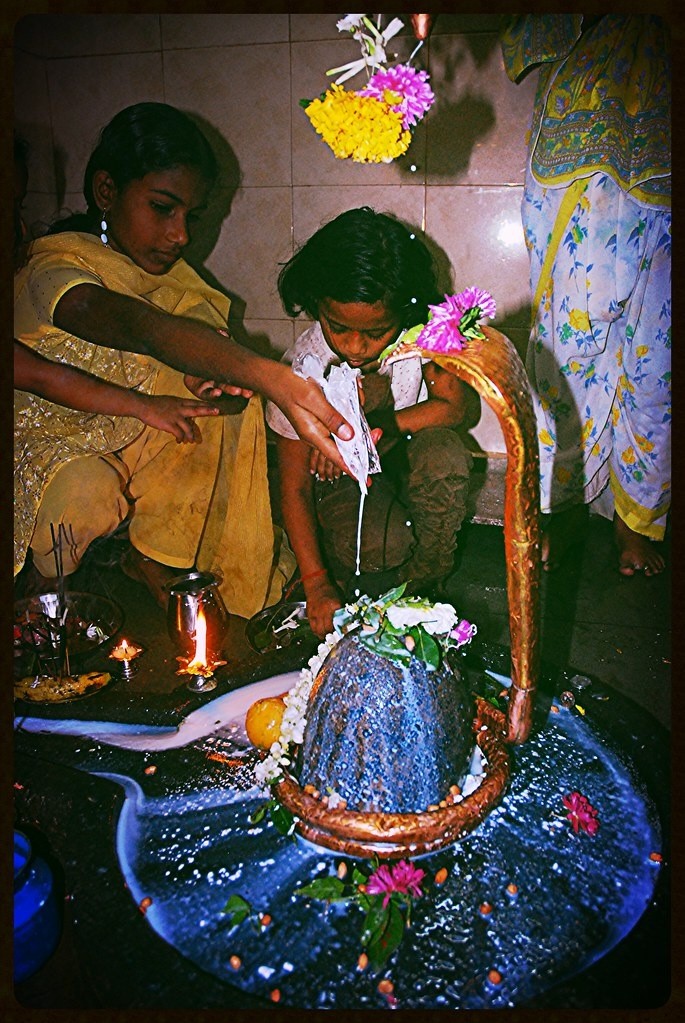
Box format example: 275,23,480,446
250,578,475,790
294,851,426,966
549,791,600,833
298,14,436,164
379,286,497,361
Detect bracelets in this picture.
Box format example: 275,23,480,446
300,569,328,581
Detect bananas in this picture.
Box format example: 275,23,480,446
12,672,113,701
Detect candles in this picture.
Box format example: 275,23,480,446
114,645,136,659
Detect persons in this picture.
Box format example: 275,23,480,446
269,205,480,641
13,339,220,444
13,103,382,621
499,0,685,578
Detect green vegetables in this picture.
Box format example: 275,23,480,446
225,580,506,963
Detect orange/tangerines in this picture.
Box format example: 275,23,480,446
245,692,289,750
